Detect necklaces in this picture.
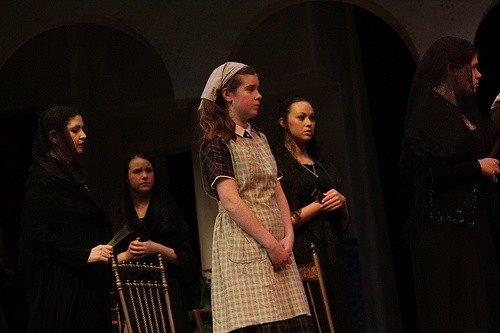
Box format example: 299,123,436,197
296,154,318,178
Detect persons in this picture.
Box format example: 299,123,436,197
197,62,314,333
403,36,500,333
268,96,352,333
17,105,193,333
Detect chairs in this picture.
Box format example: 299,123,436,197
110,252,175,333
296,242,335,333
